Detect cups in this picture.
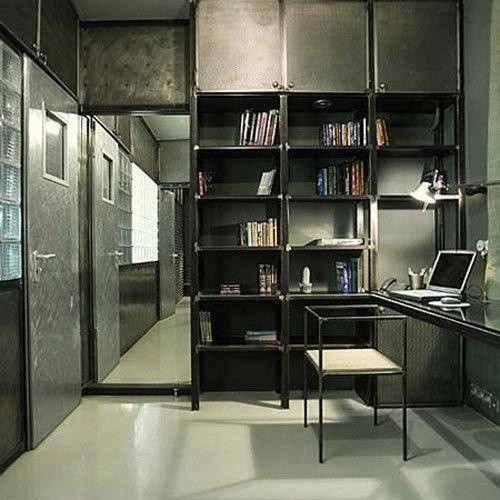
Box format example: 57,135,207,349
407,273,423,289
198,171,214,196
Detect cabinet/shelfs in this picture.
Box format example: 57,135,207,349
0,0,79,97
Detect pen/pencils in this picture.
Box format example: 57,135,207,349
407,266,427,277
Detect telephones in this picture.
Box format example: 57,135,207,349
380,277,408,293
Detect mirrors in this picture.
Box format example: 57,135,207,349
83,109,194,393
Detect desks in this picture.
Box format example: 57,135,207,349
369,289,500,408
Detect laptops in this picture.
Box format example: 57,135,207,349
390,250,478,303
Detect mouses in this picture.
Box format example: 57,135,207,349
440,297,461,304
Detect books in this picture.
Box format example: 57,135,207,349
331,257,363,293
239,108,280,145
247,330,276,335
220,283,240,295
257,168,276,195
245,335,277,341
375,118,389,147
199,171,215,197
237,215,279,247
315,238,363,246
259,264,279,293
198,310,215,343
316,117,368,196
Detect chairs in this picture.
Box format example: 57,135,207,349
300,303,411,464
